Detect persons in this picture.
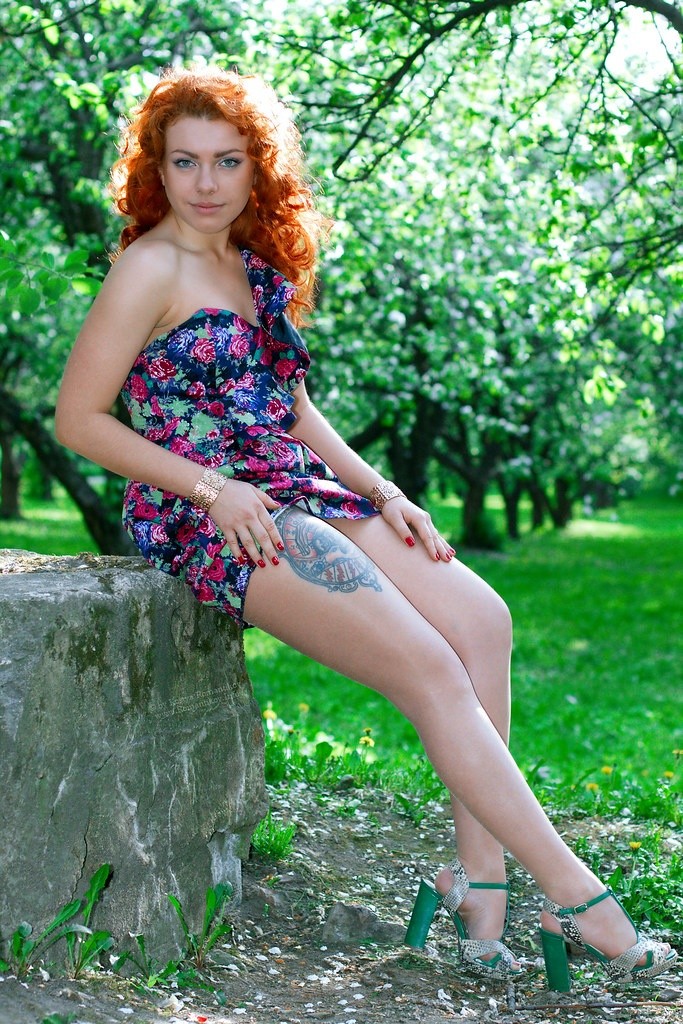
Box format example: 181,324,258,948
52,68,679,994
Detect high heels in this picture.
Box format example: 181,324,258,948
402,860,523,982
540,885,677,991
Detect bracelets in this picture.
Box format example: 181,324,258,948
189,466,227,512
368,480,407,511
